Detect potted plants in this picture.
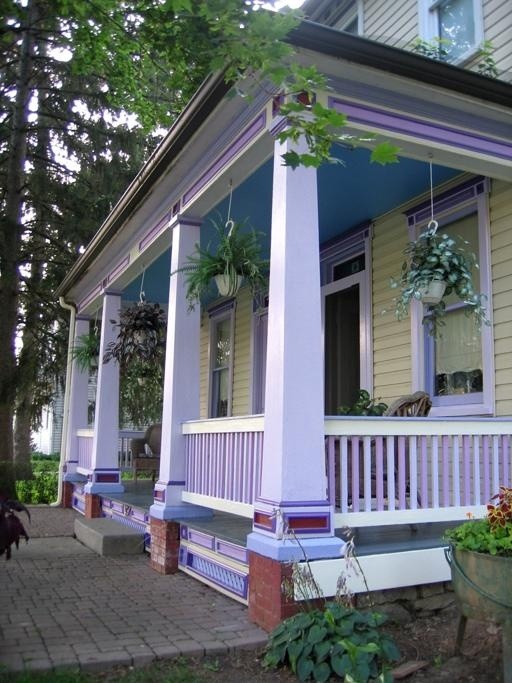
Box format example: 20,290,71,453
168,208,268,314
69,335,100,374
382,230,489,341
103,304,166,430
442,487,512,623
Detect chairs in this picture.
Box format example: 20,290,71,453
130,423,162,486
325,390,432,531
